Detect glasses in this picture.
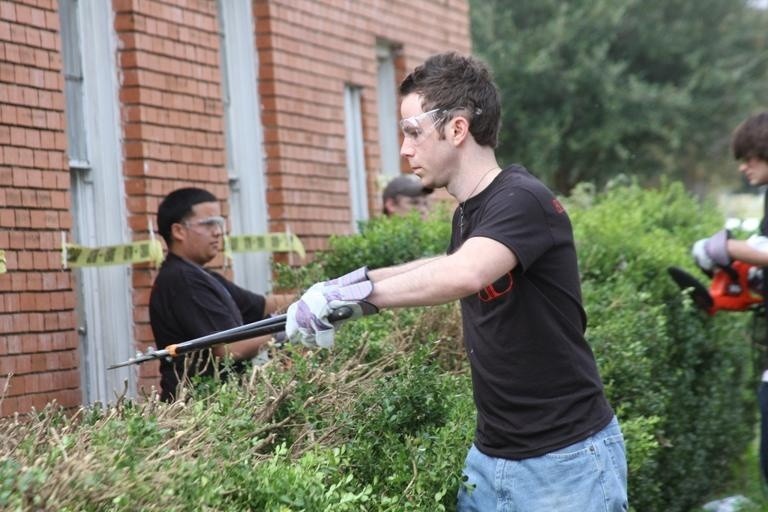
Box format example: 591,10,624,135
178,216,223,232
399,103,483,145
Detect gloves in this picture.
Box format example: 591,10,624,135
690,229,731,271
284,265,380,349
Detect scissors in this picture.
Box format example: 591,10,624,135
108,307,353,370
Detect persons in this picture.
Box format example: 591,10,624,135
380,174,433,220
688,108,768,491
282,52,632,511
148,187,301,404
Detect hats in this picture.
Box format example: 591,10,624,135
384,173,435,197
